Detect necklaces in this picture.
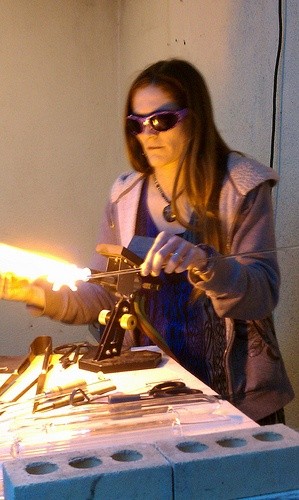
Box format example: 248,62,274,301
151,171,187,223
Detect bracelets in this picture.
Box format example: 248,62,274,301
190,243,217,276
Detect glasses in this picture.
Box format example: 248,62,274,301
125,107,193,136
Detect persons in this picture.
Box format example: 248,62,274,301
0,59,295,426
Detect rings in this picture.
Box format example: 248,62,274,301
173,250,185,261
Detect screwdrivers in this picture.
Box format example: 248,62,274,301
0,380,87,411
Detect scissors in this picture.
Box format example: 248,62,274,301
137,381,203,400
54,343,91,369
